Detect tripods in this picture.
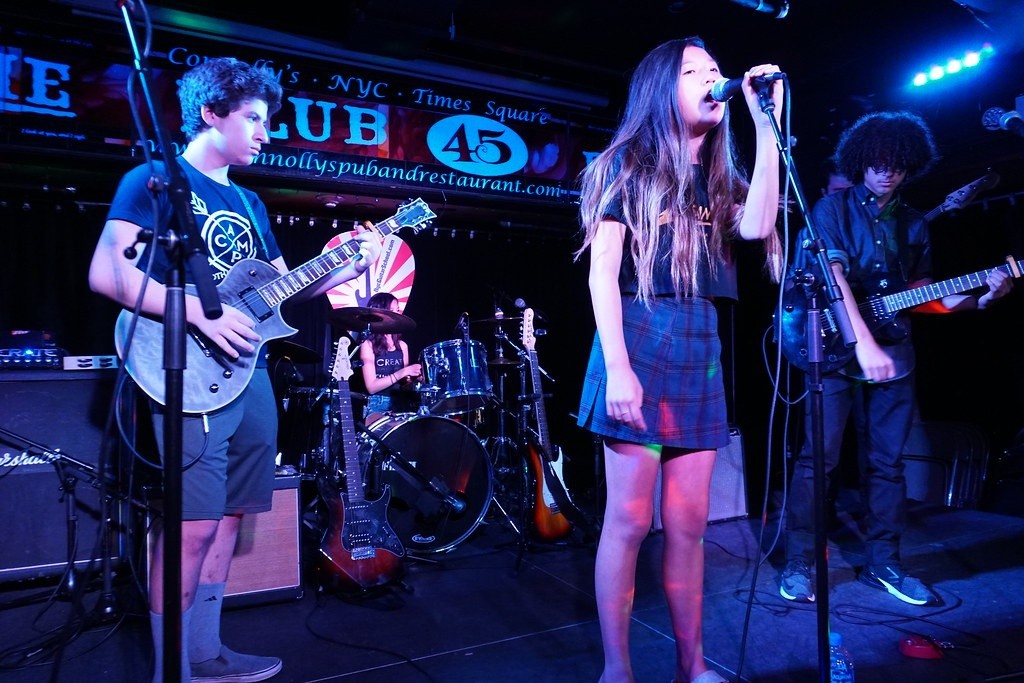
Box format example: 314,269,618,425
490,321,564,577
1,427,164,659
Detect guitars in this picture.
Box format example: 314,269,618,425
771,259,1024,374
314,334,406,588
113,197,441,421
515,308,578,537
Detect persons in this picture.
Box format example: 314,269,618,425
576,36,784,683
779,108,1014,605
359,292,426,427
88,57,384,683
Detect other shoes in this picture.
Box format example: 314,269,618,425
189,645,281,683
670,670,729,683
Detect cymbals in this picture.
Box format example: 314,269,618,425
486,356,518,369
323,306,418,336
479,315,523,323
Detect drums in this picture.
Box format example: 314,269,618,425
421,337,496,417
355,410,495,555
287,386,369,462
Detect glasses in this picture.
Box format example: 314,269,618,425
871,159,907,173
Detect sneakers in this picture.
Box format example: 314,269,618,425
779,559,816,602
858,564,940,605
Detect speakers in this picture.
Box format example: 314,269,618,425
164,473,304,615
0,367,146,580
650,428,749,534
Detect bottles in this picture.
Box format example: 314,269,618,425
830,633,854,683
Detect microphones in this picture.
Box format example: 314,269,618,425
712,72,785,103
447,496,466,514
515,298,543,319
456,312,467,332
1000,110,1024,136
